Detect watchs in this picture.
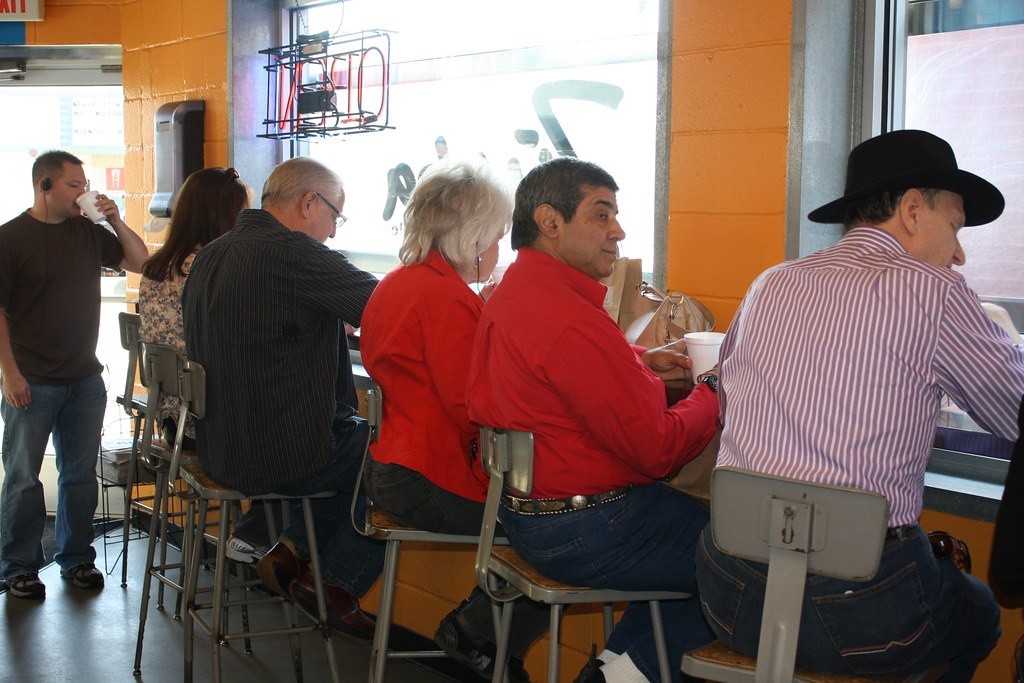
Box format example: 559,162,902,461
699,375,719,394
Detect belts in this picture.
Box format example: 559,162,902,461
498,487,631,516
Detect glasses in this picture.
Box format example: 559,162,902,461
301,191,347,228
925,530,973,572
218,167,240,201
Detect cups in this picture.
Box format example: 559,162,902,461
492,266,508,285
684,332,726,385
75,191,106,224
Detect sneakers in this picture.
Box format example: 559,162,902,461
257,543,305,600
62,563,104,588
5,570,45,597
432,608,531,683
288,566,377,647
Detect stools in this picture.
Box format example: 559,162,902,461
116,311,957,683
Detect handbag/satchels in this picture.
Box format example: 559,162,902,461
633,281,718,379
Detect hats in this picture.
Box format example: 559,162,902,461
808,129,1006,228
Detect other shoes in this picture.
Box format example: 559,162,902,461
571,642,607,683
224,534,262,566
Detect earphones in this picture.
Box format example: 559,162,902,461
43,177,51,190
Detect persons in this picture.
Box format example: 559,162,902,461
693,128,1024,683
181,157,383,642
359,164,569,683
0,150,149,598
468,158,720,683
139,168,285,562
